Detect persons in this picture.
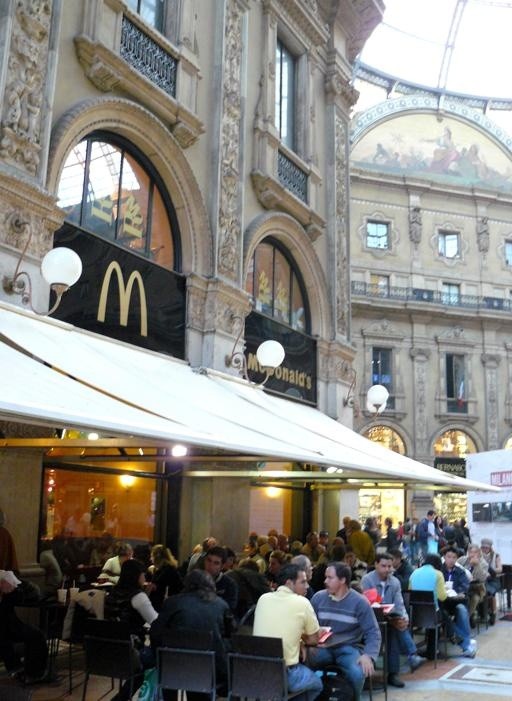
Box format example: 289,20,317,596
194,546,239,615
89,533,114,566
479,538,503,628
387,549,414,603
303,562,382,700
364,510,470,557
311,546,347,593
217,560,271,615
149,569,237,700
250,564,324,700
40,535,64,584
147,544,185,605
103,558,159,701
96,543,140,586
457,543,489,623
359,552,426,687
440,546,479,659
407,555,447,660
342,548,367,593
372,126,491,181
1,569,49,682
187,517,365,564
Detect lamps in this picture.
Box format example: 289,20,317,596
225,312,286,386
341,360,389,423
5,207,84,317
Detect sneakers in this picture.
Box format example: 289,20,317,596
464,648,474,658
410,655,427,673
489,612,496,626
388,674,406,689
470,639,479,650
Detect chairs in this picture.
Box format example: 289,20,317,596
47,603,65,667
227,636,287,699
499,566,512,608
71,604,89,694
366,609,394,700
402,587,441,668
84,619,137,701
151,628,220,700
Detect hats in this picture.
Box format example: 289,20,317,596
480,538,493,550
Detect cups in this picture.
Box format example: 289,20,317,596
70,588,79,601
446,581,454,589
57,589,67,603
